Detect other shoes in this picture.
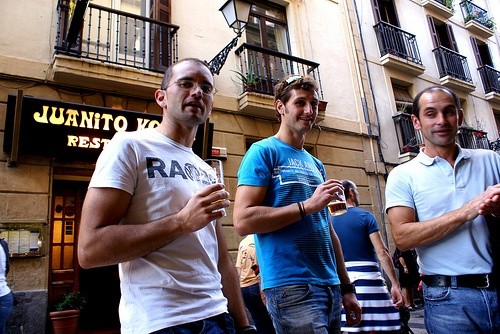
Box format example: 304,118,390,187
407,305,413,310
414,305,419,308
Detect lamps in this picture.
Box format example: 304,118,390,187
203,0,253,76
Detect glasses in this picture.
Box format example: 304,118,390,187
280,75,304,98
163,80,219,96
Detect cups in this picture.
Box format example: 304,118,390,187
198,158,228,217
326,189,348,217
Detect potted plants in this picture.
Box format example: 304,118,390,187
49,287,86,334
229,63,261,92
401,137,419,153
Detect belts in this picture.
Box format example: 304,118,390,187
420,273,495,291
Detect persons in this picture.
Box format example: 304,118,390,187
77,58,256,334
383,86,500,334
235,234,276,334
232,74,361,334
0,239,13,334
395,247,423,310
329,180,404,334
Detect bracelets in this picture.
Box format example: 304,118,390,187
237,325,257,334
341,284,356,295
297,202,306,219
405,267,407,268
